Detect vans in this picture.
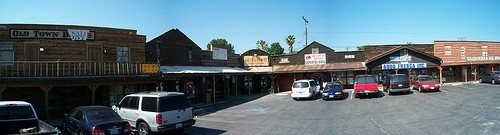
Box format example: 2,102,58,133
383,74,411,94
354,75,379,98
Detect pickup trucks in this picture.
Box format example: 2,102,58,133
0,100,63,135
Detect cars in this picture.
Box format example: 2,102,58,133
413,74,441,93
63,105,132,135
478,71,500,84
322,81,345,100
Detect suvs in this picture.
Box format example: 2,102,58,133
111,91,196,135
291,78,320,100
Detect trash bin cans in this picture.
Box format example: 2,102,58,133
271,85,279,93
